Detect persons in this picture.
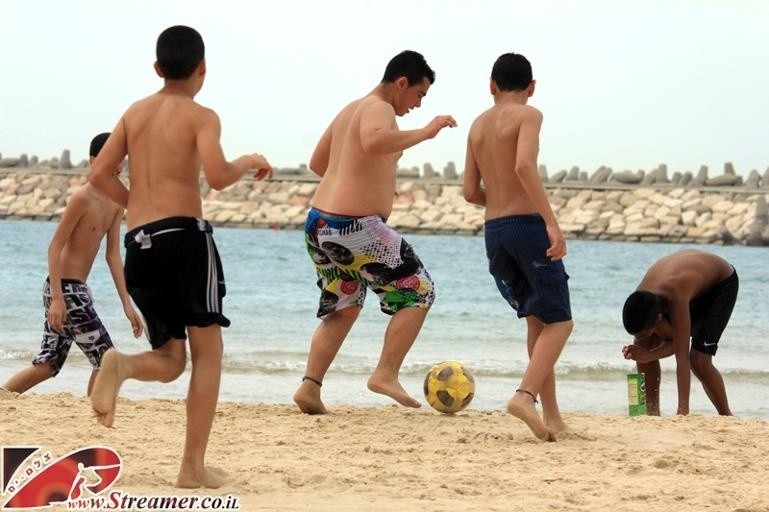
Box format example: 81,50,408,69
622,247,739,417
294,50,458,414
1,131,144,396
462,51,575,442
88,23,274,488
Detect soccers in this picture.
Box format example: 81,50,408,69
424,360,475,414
